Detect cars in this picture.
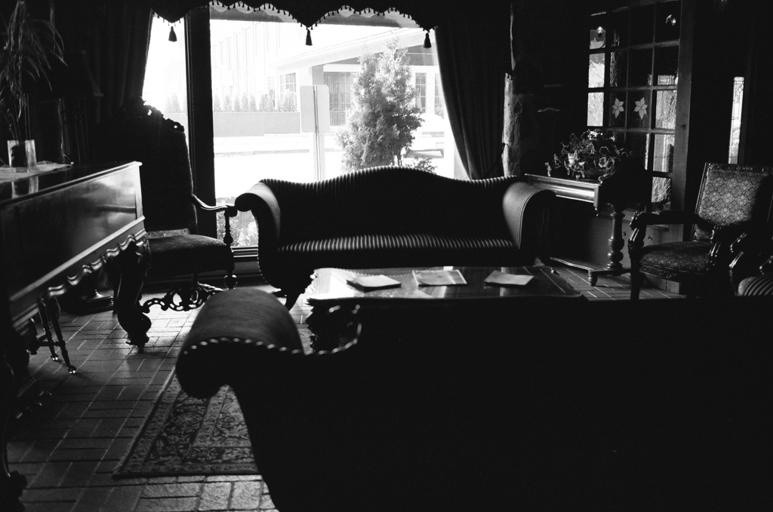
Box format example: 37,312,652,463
399,114,447,159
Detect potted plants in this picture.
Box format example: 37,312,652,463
0,0,70,166
546,126,632,184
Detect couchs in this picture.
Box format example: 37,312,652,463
234,166,561,311
174,287,773,512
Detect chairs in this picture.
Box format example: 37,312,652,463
78,93,237,346
627,160,773,300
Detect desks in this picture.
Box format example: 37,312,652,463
523,159,652,286
0,160,151,512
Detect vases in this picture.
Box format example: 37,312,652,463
11,176,39,199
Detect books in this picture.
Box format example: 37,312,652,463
347,274,401,292
412,269,468,287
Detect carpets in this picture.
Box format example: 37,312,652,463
112,368,260,480
